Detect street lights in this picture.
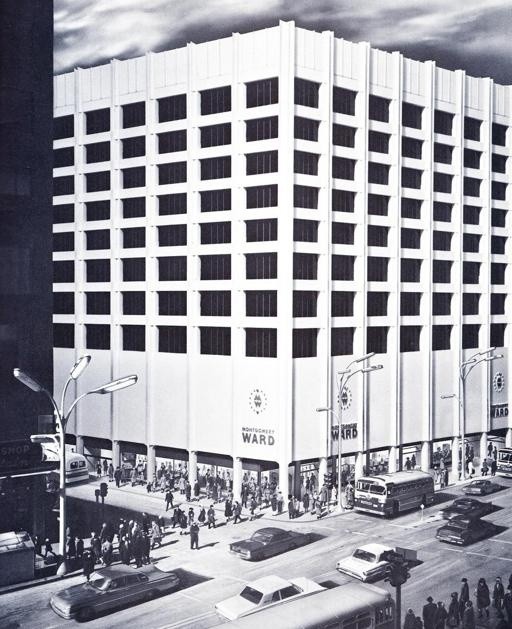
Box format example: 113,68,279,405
14,355,137,577
316,351,384,512
440,346,504,480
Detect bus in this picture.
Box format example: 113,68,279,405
496,448,512,479
204,582,396,629
355,470,434,517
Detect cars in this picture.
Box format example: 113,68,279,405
229,526,308,560
463,479,501,495
336,544,397,583
215,576,327,623
437,516,496,547
439,498,494,521
48,563,179,623
30,434,90,491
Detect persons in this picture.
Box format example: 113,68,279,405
403,575,512,629
35,442,512,581
